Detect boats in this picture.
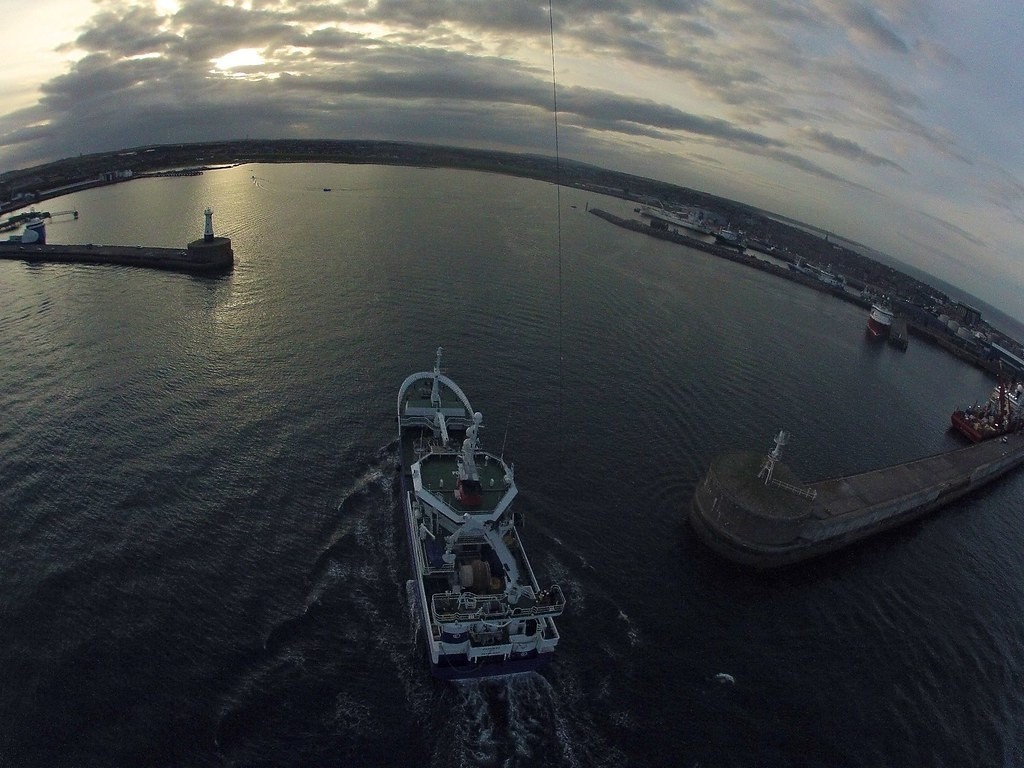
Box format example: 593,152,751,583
788,259,844,289
712,222,747,250
950,360,1024,445
396,347,568,679
866,295,894,343
640,203,713,233
